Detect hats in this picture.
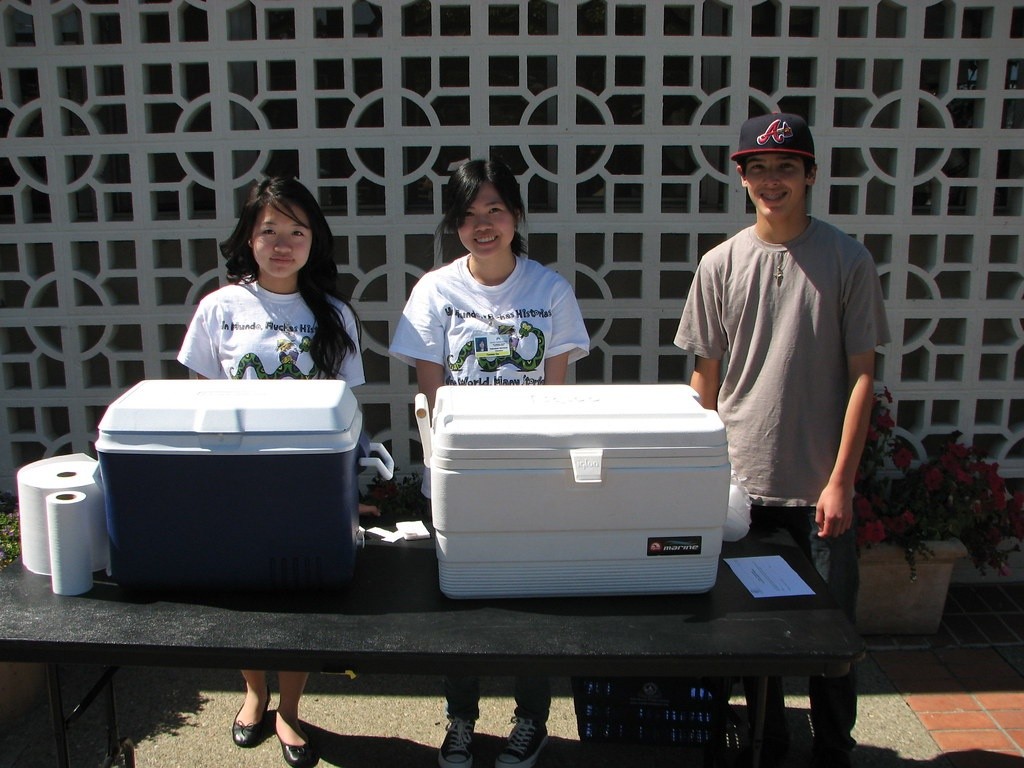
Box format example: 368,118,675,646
733,111,817,162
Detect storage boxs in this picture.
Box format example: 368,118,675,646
568,676,729,749
412,383,733,599
93,377,365,589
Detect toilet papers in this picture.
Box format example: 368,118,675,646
46,491,95,598
16,451,111,578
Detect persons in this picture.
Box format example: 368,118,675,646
385,159,592,768
477,340,487,351
673,110,891,768
175,177,367,768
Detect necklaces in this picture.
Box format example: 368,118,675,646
755,220,809,285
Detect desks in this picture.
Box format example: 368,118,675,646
0,521,867,768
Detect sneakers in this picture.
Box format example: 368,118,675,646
437,716,475,768
497,717,548,768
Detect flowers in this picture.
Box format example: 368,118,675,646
853,386,1024,584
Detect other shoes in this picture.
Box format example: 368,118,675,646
234,687,266,747
274,714,309,764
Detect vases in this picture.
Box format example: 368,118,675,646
854,541,967,635
